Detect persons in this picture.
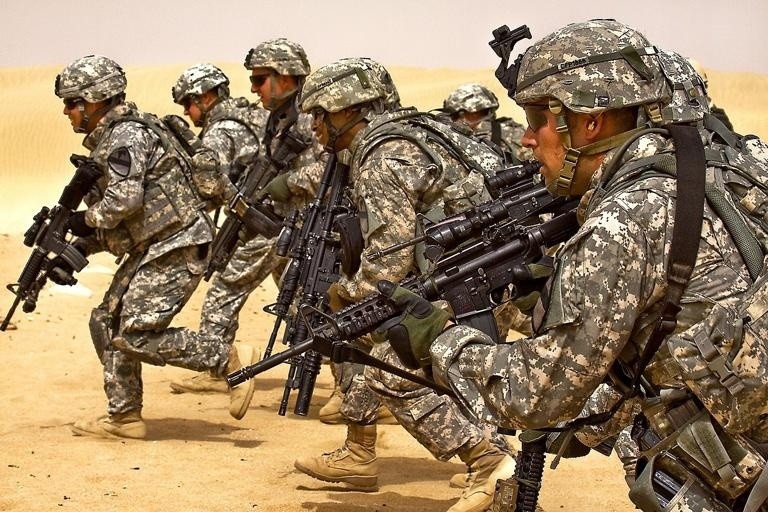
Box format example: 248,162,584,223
56,54,261,439
301,57,516,512
368,15,768,512
172,38,534,490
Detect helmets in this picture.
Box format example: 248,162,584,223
516,18,708,124
243,38,311,76
444,85,499,112
302,58,400,111
173,65,228,102
55,56,127,104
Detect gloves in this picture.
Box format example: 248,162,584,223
371,280,454,367
316,283,347,319
511,215,565,315
224,208,257,247
252,171,292,206
69,211,97,237
46,245,86,284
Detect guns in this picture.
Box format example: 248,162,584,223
262,151,341,369
204,131,310,285
0,153,104,331
490,429,555,512
276,162,362,418
223,158,582,436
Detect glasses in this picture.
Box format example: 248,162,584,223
312,107,324,119
524,104,549,131
63,97,85,108
181,97,191,111
250,74,271,87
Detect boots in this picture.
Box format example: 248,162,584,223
445,433,520,512
170,369,232,395
319,360,345,422
222,342,260,420
295,423,378,486
73,408,148,441
